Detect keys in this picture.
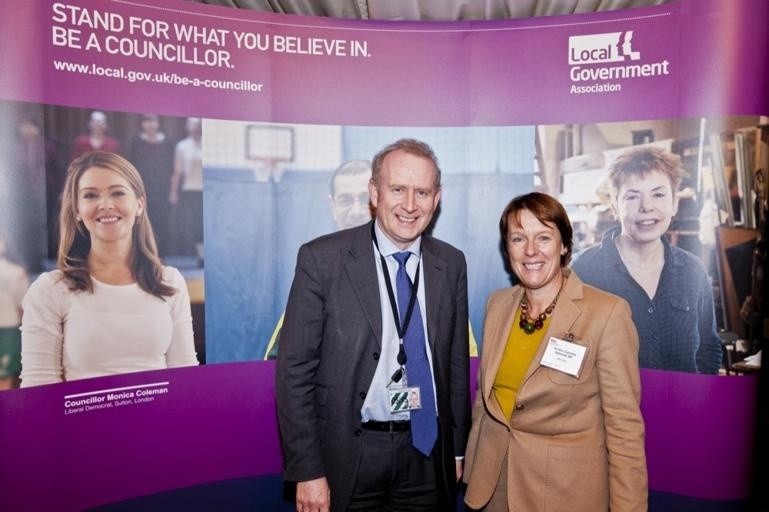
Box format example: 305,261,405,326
386,369,407,389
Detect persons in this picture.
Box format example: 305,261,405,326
264,160,478,361
18,150,201,389
277,138,471,512
10,110,203,267
462,192,649,512
569,145,723,375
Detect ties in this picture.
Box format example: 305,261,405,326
392,253,438,458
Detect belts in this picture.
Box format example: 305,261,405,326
361,421,411,432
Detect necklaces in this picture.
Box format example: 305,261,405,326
518,275,565,335
